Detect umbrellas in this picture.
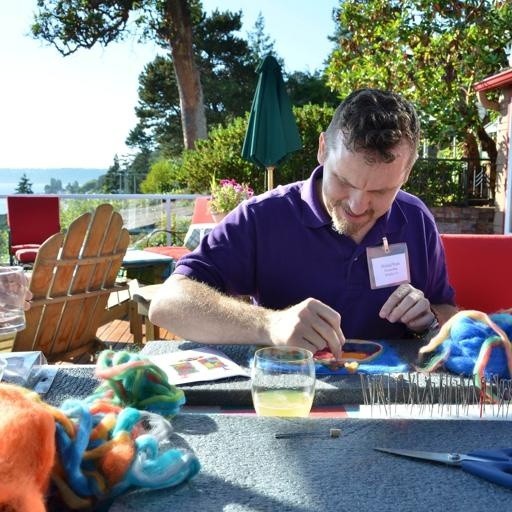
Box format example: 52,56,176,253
237,51,303,192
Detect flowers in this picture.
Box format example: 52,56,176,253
209,171,254,215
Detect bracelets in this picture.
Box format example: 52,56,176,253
410,302,440,340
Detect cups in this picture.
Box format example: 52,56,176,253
1,265,28,335
250,345,315,417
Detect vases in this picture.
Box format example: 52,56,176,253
211,214,226,224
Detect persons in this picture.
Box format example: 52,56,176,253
151,87,465,365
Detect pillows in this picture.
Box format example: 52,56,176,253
182,223,215,251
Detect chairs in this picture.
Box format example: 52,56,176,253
436,232,511,316
6,193,143,367
144,196,216,261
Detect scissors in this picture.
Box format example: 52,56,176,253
374,448,512,486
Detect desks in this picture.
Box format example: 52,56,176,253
112,245,173,342
0,358,512,510
